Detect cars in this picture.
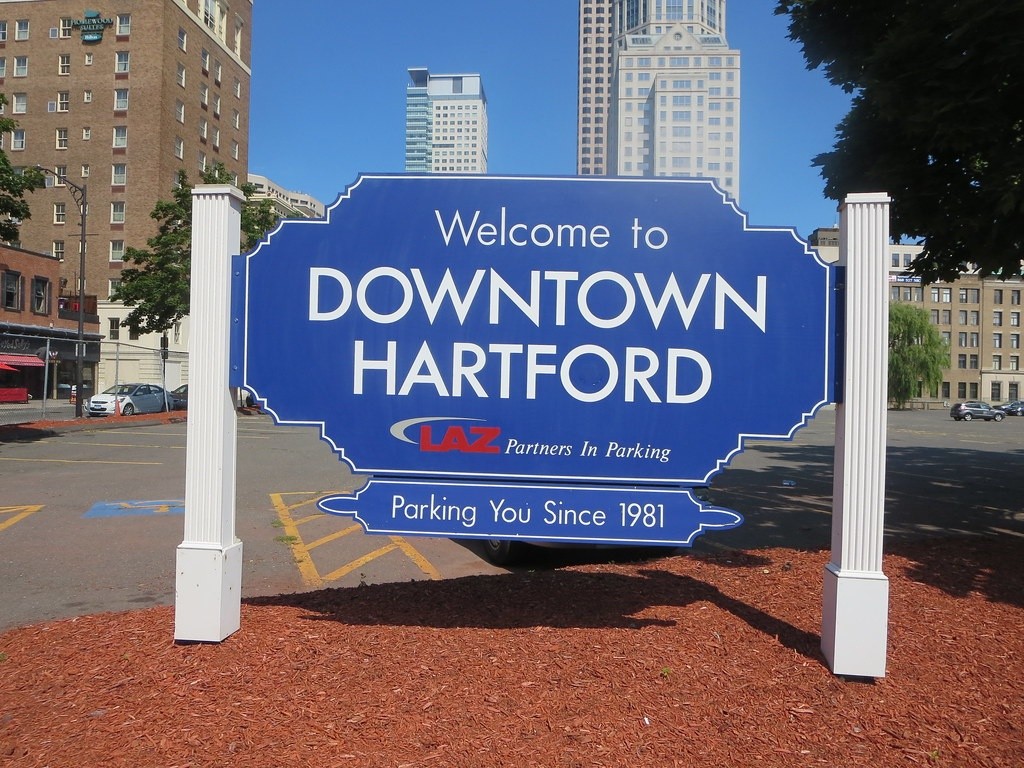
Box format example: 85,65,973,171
83,383,175,416
992,400,1024,416
170,383,188,410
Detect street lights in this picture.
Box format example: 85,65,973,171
32,165,88,417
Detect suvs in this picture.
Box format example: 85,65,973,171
950,400,1007,422
